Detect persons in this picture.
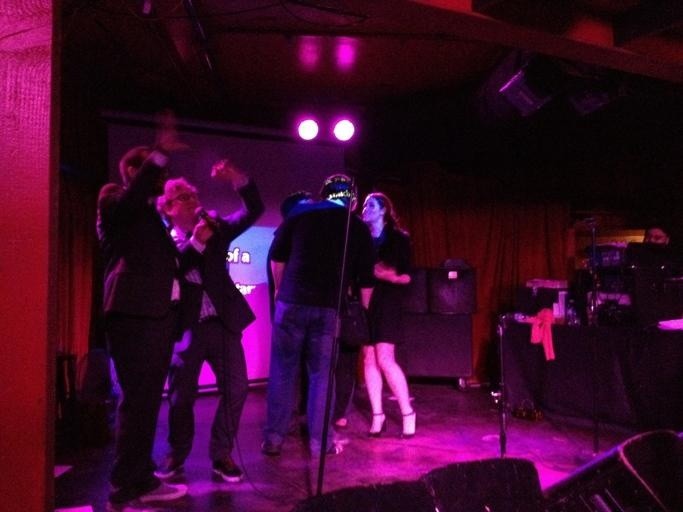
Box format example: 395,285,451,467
265,191,356,429
360,191,419,439
259,172,375,459
641,225,669,244
151,158,266,482
94,124,189,506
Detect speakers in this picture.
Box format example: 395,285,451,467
417,457,549,512
542,431,683,512
401,268,428,314
428,266,477,314
289,481,437,512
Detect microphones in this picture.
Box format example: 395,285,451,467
195,207,219,232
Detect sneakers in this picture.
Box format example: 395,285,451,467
260,440,281,456
139,481,188,503
212,459,244,482
326,417,350,454
153,462,184,478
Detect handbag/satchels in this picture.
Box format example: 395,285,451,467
340,299,369,347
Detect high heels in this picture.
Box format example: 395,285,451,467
368,412,386,438
402,410,418,440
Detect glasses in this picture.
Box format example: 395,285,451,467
170,193,198,202
